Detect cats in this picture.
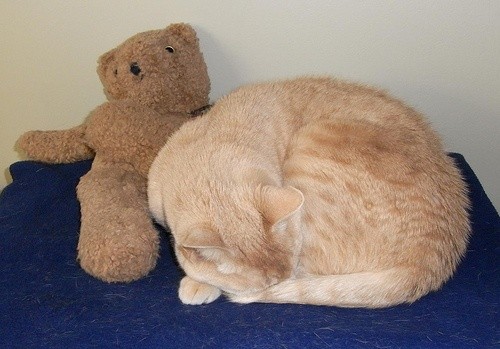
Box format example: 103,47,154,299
146,73,476,309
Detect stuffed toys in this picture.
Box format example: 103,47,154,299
14,22,213,284
